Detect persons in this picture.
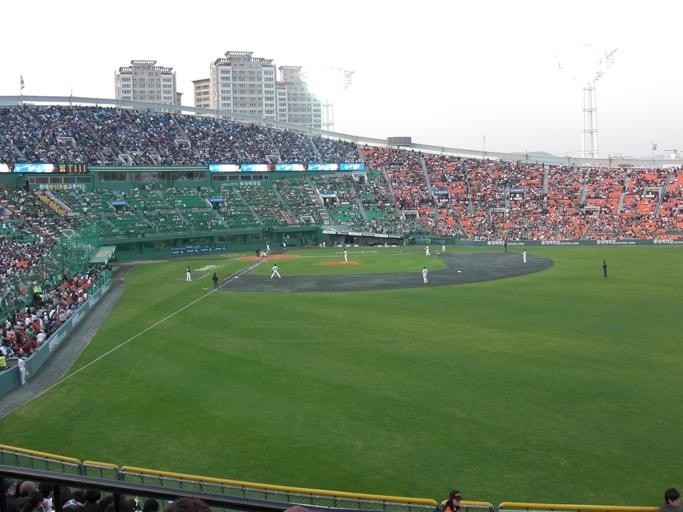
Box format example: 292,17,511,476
284,506,310,512
658,488,683,512
18,355,27,385
444,490,461,512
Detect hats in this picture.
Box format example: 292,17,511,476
449,490,463,501
18,480,40,498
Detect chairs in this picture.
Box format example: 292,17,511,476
0,103,113,375
111,108,327,259
501,154,680,247
310,128,506,247
